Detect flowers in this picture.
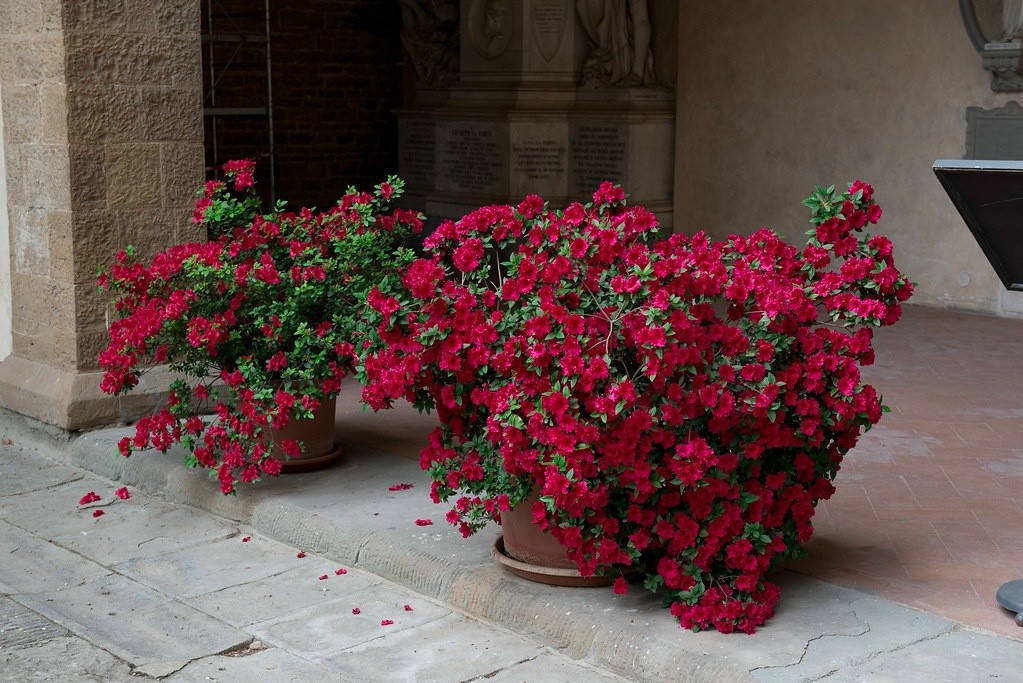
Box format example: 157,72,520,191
355,179,920,639
89,156,426,497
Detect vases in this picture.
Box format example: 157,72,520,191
490,489,620,589
243,380,343,474
